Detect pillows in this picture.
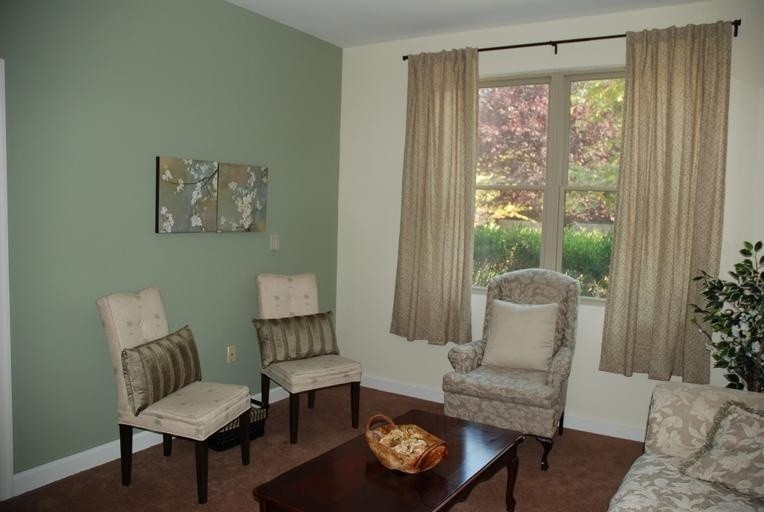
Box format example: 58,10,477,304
251,311,340,366
482,300,557,371
680,401,764,506
122,324,200,417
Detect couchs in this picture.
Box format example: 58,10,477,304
607,381,764,512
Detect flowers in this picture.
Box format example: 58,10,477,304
684,241,764,393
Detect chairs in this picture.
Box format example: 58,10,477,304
253,272,363,444
443,268,580,471
96,287,252,504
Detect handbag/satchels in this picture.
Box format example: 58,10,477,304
365,414,449,475
